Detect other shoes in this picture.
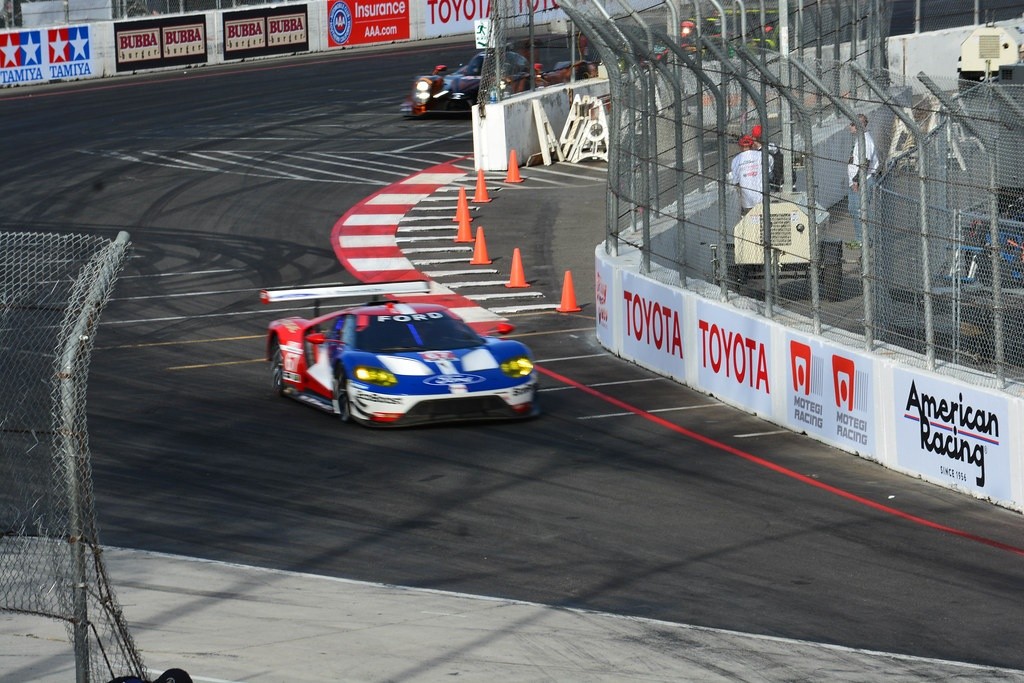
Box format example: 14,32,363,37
844,240,862,249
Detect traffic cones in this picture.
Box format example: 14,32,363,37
470,169,492,202
469,226,493,265
451,186,474,222
503,148,524,183
505,247,531,288
453,204,476,243
553,270,593,313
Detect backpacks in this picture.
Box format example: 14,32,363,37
758,143,797,185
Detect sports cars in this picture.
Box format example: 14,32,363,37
257,277,542,431
538,3,786,86
400,48,552,119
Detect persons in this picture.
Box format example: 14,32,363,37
847,114,879,249
732,126,779,219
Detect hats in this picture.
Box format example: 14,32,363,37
738,136,754,146
752,126,762,137
